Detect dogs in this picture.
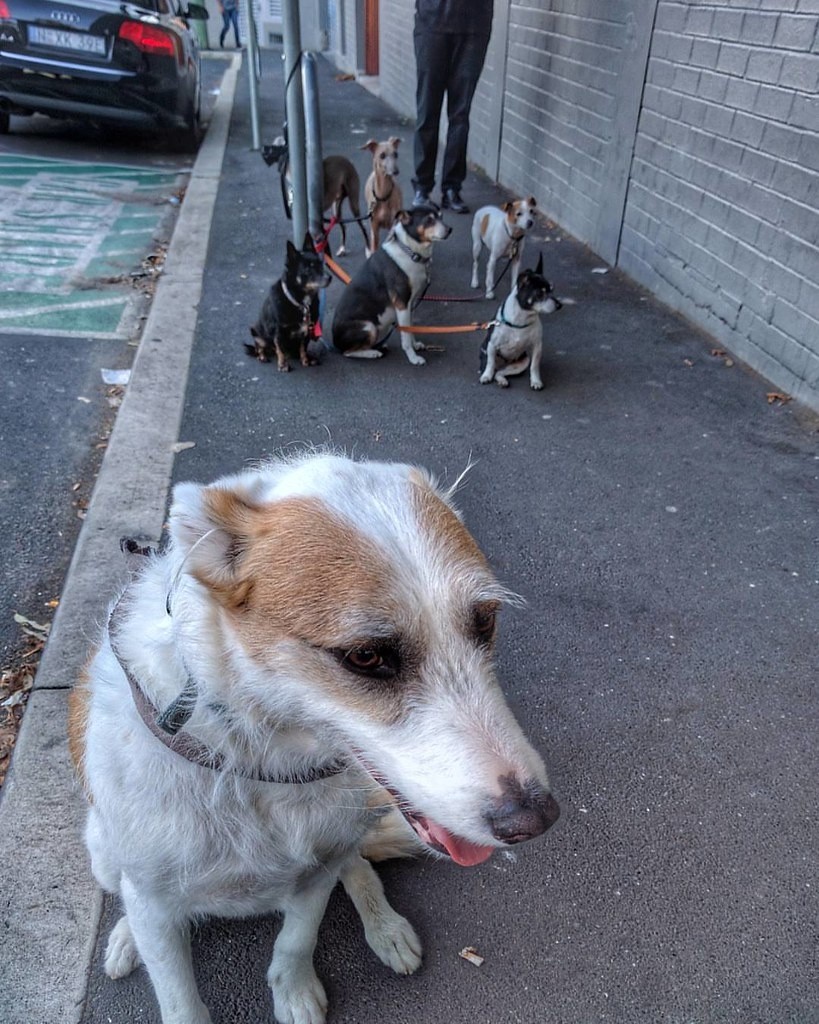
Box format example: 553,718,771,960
477,268,564,392
331,199,454,367
261,143,372,260
356,136,404,255
470,195,538,301
65,449,562,1024
244,229,333,373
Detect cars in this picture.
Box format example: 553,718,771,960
0,1,212,154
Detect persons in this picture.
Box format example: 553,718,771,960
412,0,494,215
217,0,241,48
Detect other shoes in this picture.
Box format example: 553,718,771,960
236,44,241,48
220,41,223,48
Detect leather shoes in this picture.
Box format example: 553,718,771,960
412,190,427,207
442,189,468,213
